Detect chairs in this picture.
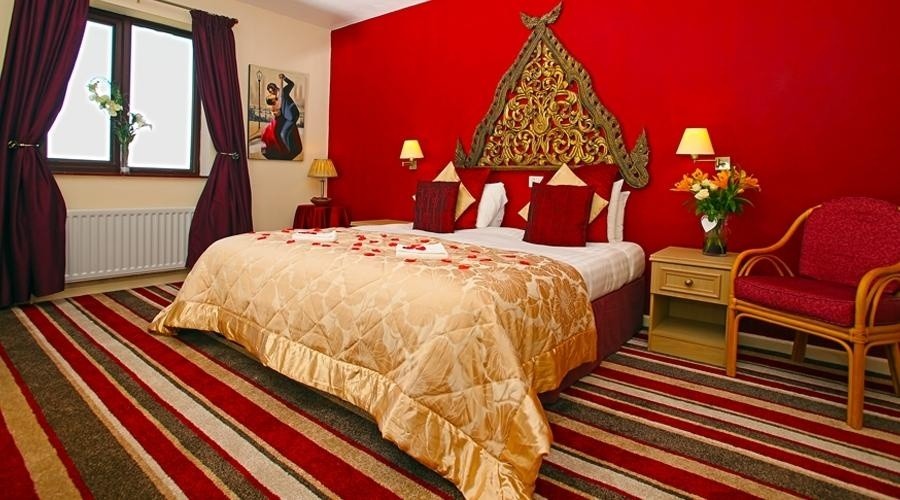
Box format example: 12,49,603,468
728,196,900,431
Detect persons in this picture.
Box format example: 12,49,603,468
261,74,302,161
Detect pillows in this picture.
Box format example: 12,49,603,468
450,168,491,228
607,176,624,247
476,181,509,229
412,180,461,234
517,161,609,230
410,160,476,226
522,182,595,247
617,190,632,242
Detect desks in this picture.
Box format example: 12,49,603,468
292,204,351,228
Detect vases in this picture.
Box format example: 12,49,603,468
703,223,726,258
119,143,129,176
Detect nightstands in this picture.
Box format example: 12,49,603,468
350,218,411,227
646,246,740,368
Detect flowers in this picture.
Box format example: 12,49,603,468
670,166,761,255
87,78,153,165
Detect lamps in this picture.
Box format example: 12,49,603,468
675,128,731,171
307,159,338,206
399,139,425,171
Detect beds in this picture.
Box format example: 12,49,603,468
147,2,647,498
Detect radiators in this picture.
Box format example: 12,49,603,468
66,205,195,282
